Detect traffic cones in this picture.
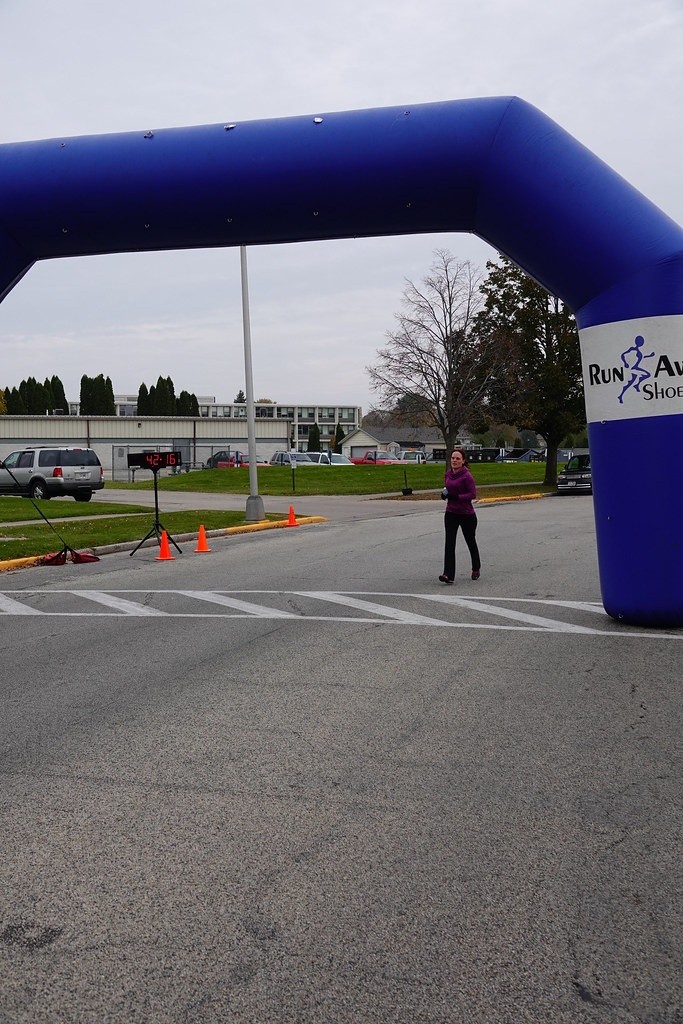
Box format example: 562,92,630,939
154,530,176,560
193,525,212,552
285,505,300,527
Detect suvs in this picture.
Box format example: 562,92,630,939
0,445,105,502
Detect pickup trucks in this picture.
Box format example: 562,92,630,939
217,453,272,467
347,450,400,465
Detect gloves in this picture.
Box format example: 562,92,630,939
446,494,458,501
441,493,448,500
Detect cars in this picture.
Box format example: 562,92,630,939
395,451,427,464
556,453,594,495
270,450,314,466
306,452,356,465
206,450,244,469
421,452,447,464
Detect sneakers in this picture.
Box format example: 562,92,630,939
439,573,454,583
472,568,480,580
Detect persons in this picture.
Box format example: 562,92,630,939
439,449,480,584
